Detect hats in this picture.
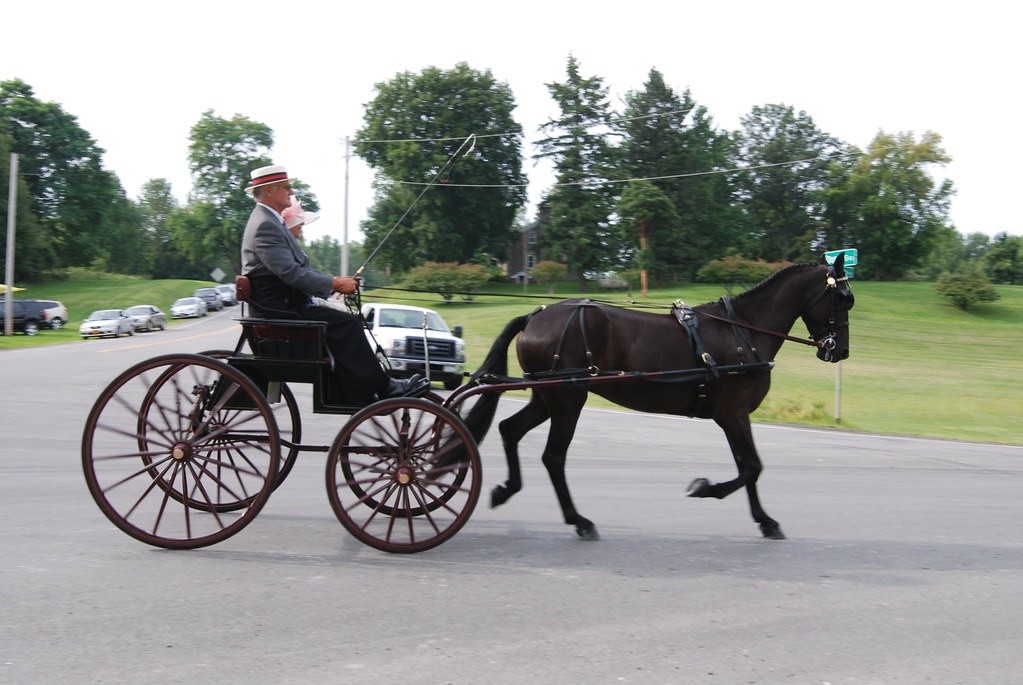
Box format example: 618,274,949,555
282,201,320,231
244,165,300,194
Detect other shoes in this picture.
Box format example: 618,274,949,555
376,373,421,400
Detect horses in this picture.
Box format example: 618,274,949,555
428,252,854,540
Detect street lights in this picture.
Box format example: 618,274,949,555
340,136,378,277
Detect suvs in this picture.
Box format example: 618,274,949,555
0,299,47,336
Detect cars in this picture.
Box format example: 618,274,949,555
79,309,136,339
215,285,237,306
225,283,236,290
190,287,224,311
357,300,466,391
307,297,358,316
125,305,167,331
35,299,69,330
170,297,208,319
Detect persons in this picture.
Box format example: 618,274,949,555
242,165,420,399
282,195,318,238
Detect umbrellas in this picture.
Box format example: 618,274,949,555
0,285,27,295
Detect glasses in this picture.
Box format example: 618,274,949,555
268,183,292,192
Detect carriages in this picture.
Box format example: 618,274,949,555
81,249,856,550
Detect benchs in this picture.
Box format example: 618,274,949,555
220,246,337,376
